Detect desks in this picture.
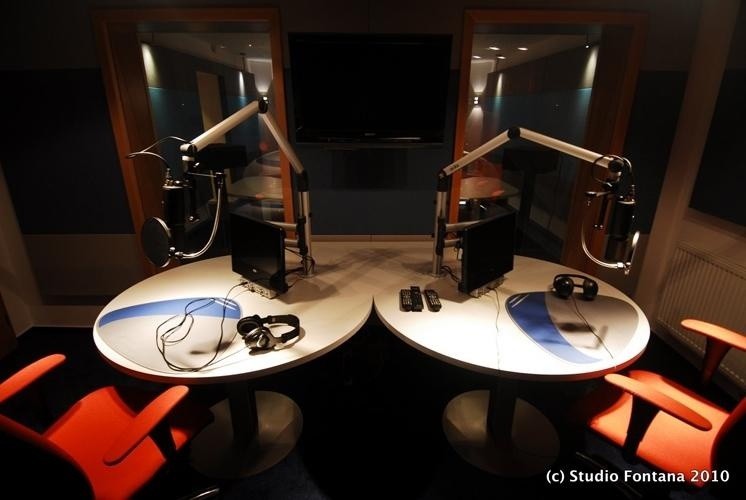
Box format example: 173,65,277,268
226,176,283,220
459,176,520,221
374,246,651,479
91,246,374,480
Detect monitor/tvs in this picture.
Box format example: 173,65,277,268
288,31,453,144
460,212,514,294
226,210,287,295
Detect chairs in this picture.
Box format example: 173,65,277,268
463,150,500,180
0,352,216,500
568,318,746,500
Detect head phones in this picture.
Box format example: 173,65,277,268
237,314,300,351
552,272,600,301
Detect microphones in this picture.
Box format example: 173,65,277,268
160,184,189,255
603,198,637,262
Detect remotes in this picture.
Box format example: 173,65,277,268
425,288,441,311
400,287,412,311
410,285,423,311
